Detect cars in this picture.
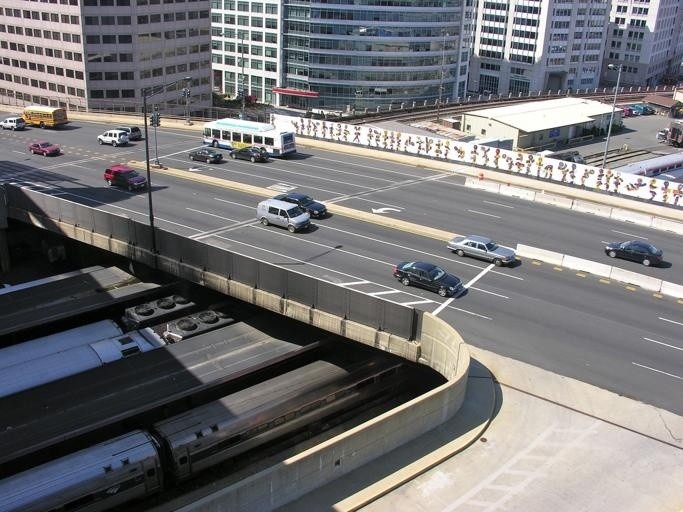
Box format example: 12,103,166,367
391,260,461,296
187,148,222,164
115,125,141,140
446,233,516,269
27,140,60,156
214,86,220,93
273,191,328,218
620,104,655,119
604,239,663,268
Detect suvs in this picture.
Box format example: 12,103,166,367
0,117,25,131
102,165,146,193
95,130,128,149
228,144,269,163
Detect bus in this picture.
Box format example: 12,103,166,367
201,116,297,158
22,105,69,130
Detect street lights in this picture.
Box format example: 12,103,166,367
437,30,450,120
219,32,247,114
601,64,624,168
142,76,193,230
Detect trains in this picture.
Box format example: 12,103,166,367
536,149,587,167
610,153,683,177
650,168,683,184
666,120,683,147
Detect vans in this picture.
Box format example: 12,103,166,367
256,197,313,234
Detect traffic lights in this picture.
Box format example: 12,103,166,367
149,114,154,126
156,113,160,127
186,91,190,99
181,91,184,97
